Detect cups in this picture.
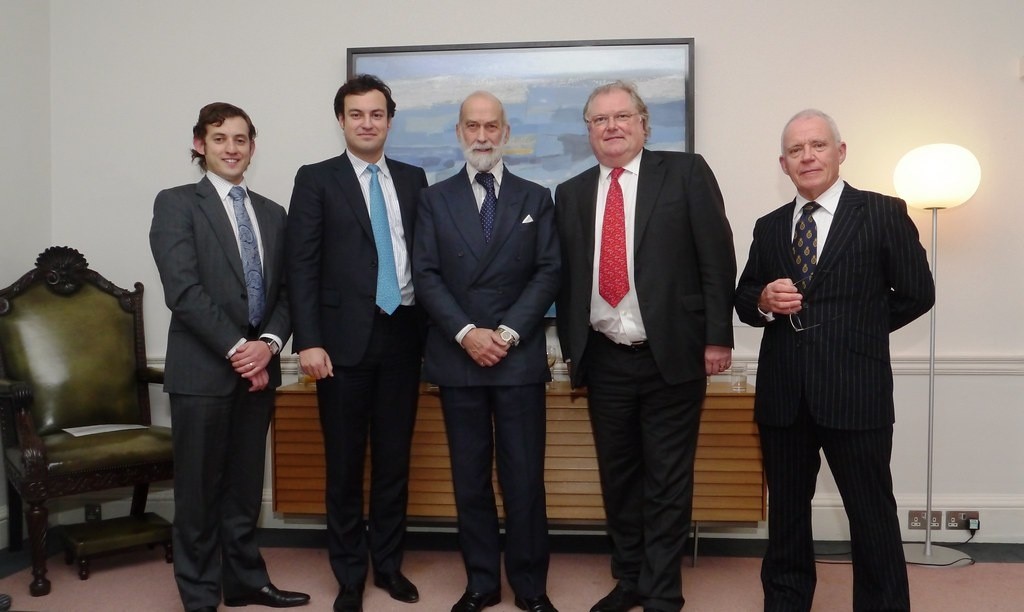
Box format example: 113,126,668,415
297,357,305,384
305,375,316,391
731,363,747,391
546,346,556,368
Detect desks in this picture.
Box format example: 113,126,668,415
270,383,767,569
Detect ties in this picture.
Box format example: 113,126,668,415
792,200,822,293
367,164,401,316
228,186,265,327
599,168,630,309
474,173,499,246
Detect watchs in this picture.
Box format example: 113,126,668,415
497,327,516,347
259,337,279,357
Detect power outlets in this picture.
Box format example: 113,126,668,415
946,511,980,530
908,510,942,531
85,504,102,523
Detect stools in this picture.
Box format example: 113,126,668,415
60,511,174,580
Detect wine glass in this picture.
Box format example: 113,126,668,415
546,346,559,384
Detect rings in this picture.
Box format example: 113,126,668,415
480,362,484,367
249,363,253,369
719,364,725,368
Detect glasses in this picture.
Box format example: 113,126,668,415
587,111,641,128
463,121,502,133
790,269,845,332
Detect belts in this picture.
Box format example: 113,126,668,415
375,306,412,316
618,340,648,351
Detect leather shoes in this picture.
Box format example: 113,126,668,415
185,607,217,612
515,595,559,612
451,584,502,612
333,584,365,612
589,584,642,612
643,596,685,612
374,569,419,603
224,583,310,607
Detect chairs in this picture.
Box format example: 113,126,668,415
0,246,177,598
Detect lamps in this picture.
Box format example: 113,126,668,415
892,142,982,569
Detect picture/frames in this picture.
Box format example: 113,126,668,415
346,36,694,326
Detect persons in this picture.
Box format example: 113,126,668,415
288,73,427,612
412,92,563,612
554,81,738,612
734,109,936,612
150,103,311,612
